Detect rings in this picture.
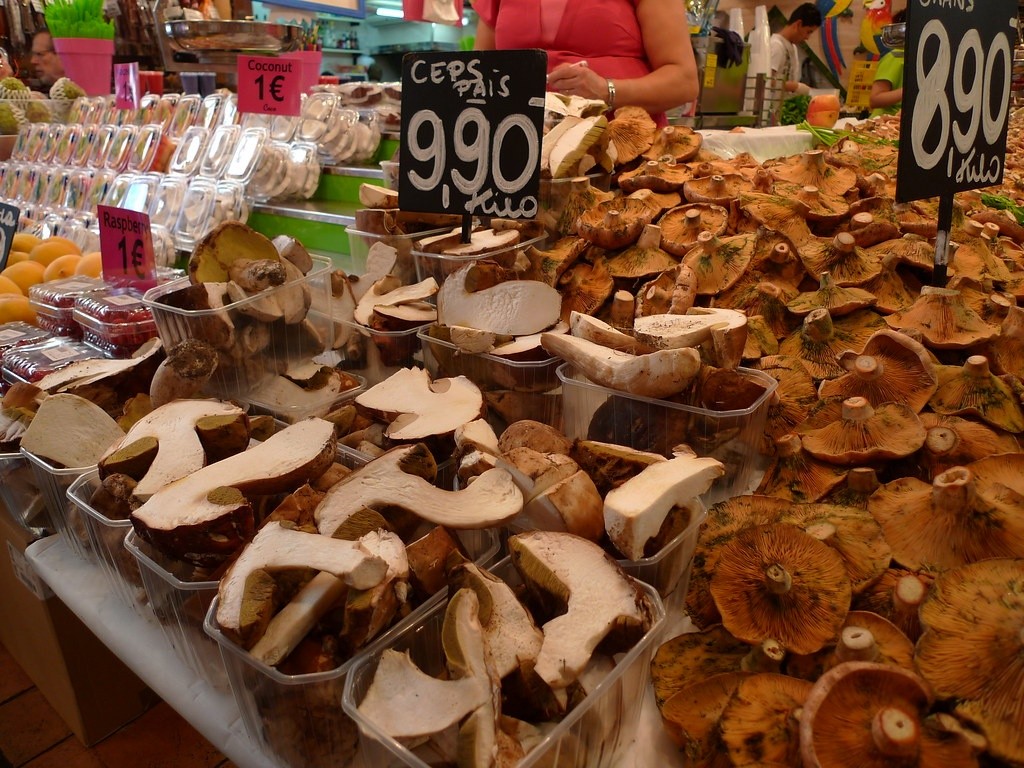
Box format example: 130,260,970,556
566,90,568,95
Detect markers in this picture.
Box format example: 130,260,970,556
546,59,589,84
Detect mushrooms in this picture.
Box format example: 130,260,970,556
513,104,1024,768
0,82,773,768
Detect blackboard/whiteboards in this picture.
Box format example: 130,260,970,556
398,47,548,222
895,0,1018,205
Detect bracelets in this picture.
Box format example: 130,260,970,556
605,78,616,113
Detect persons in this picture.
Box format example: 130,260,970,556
867,6,904,121
768,2,827,124
470,0,700,136
0,27,65,100
